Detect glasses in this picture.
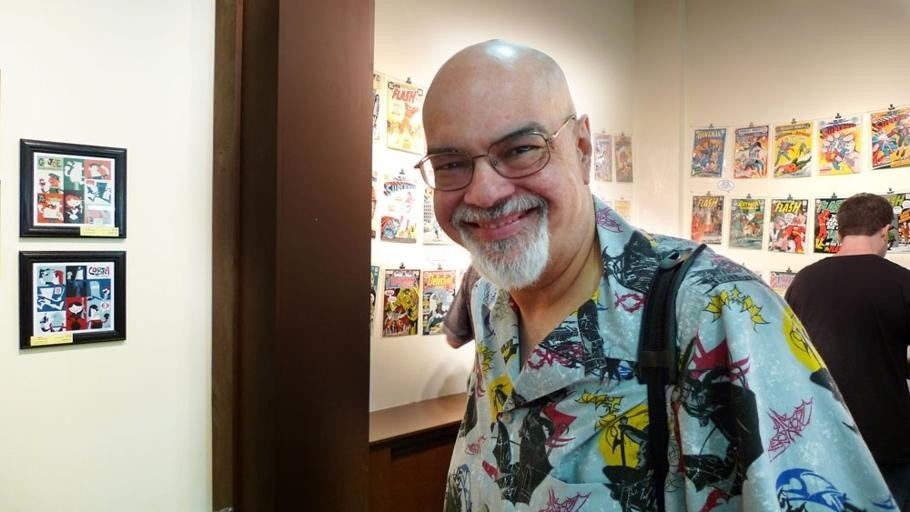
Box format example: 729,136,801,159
414,115,574,192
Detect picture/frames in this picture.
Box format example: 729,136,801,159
19,141,127,344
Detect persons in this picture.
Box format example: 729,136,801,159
413,38,902,512
783,193,910,512
443,265,482,348
